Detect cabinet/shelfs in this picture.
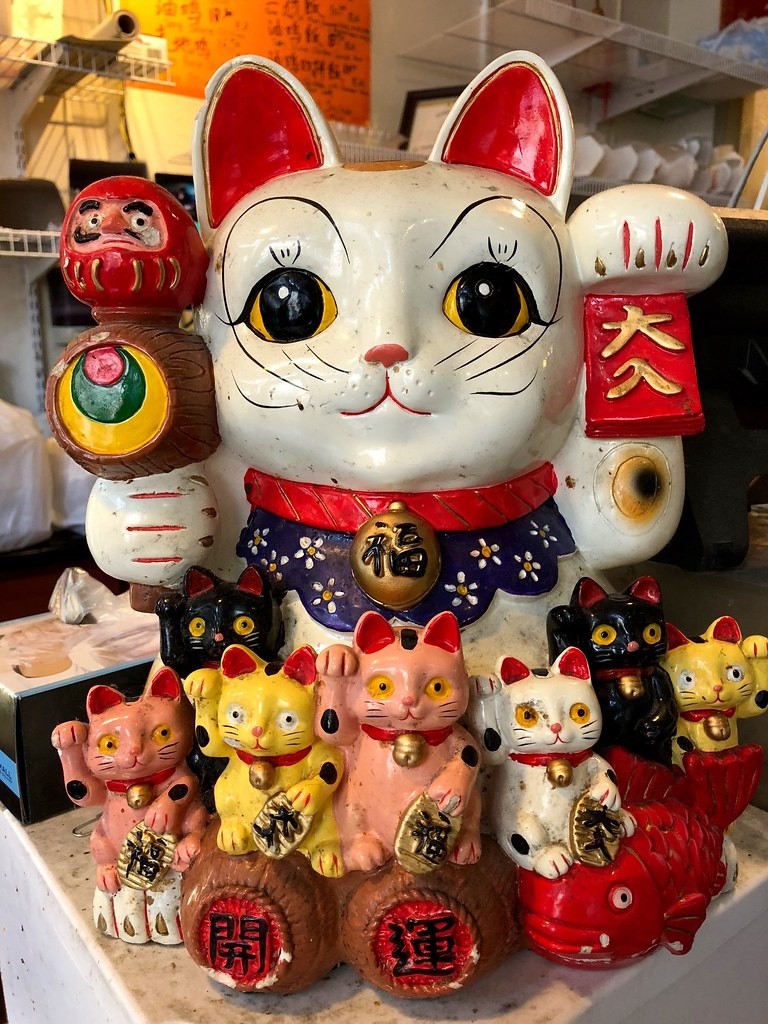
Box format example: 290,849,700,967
0,33,176,413
399,0,768,213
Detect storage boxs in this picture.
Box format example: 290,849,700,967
0,593,160,825
